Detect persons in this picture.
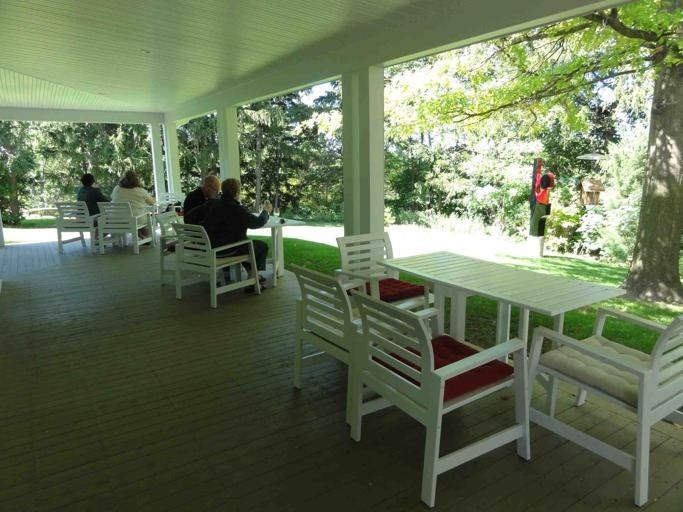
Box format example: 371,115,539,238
204,177,272,291
183,175,239,253
527,173,554,236
78,173,110,226
109,170,156,247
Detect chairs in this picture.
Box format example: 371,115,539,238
166,220,262,309
280,258,421,424
524,300,683,506
344,288,533,507
154,205,202,289
96,199,160,255
334,227,443,349
56,194,123,256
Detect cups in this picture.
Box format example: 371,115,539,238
258,205,263,213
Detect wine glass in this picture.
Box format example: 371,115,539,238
273,207,281,224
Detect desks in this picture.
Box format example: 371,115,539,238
376,251,630,434
